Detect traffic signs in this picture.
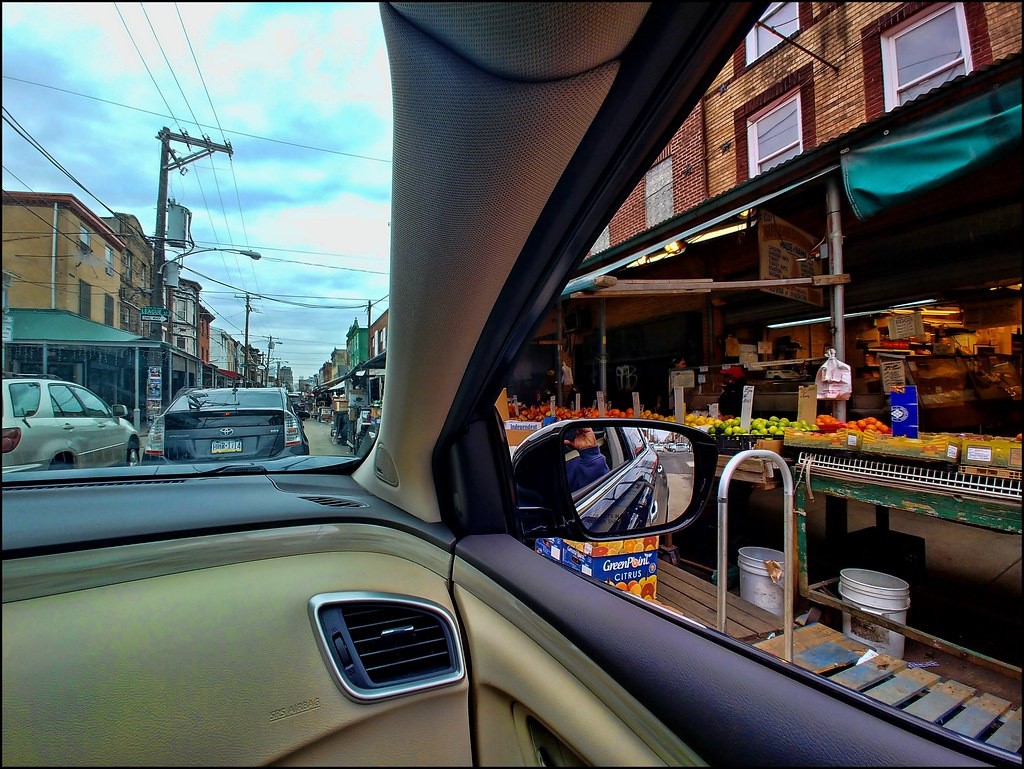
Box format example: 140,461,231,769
140,307,169,323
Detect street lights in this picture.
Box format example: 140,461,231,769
157,247,262,413
264,337,283,388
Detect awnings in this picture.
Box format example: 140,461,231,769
2,310,149,341
321,350,386,386
551,47,1024,302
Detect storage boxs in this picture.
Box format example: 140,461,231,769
710,430,825,455
495,388,540,460
821,527,926,581
534,535,659,597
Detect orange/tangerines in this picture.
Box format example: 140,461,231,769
816,414,888,434
585,408,634,418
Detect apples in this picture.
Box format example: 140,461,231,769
520,404,584,421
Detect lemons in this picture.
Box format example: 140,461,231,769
662,413,820,436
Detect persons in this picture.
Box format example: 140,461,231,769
670,357,686,370
564,427,610,493
716,367,746,418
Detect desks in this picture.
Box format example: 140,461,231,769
654,557,798,643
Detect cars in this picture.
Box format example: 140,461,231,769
649,442,676,452
672,443,688,453
285,391,309,420
654,444,665,453
141,387,309,466
1,377,142,477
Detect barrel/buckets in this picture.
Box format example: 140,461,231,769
839,568,910,660
738,546,785,617
